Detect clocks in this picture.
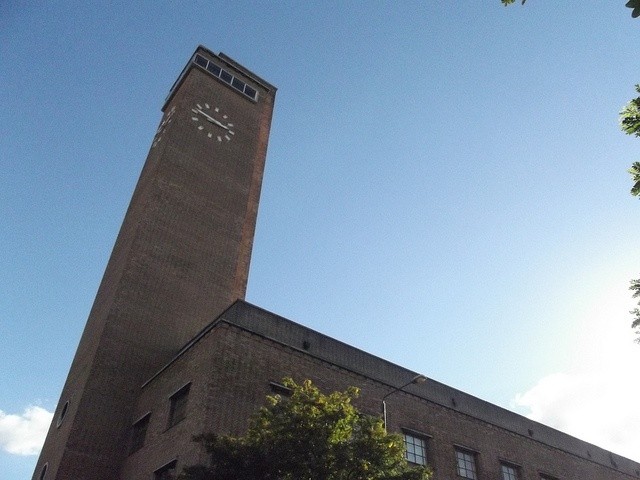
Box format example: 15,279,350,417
152,105,175,148
190,102,235,142
